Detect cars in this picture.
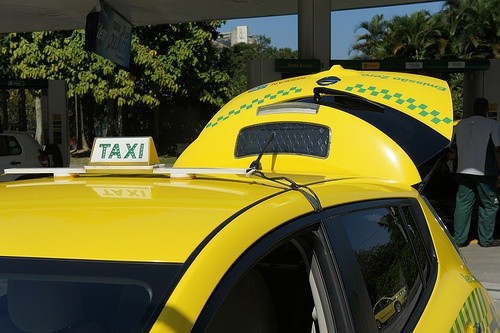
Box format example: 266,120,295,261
373,284,409,329
1,65,500,333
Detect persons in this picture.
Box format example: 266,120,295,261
452,98,500,247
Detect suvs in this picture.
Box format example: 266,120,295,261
0,129,50,183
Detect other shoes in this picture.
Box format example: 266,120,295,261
456,238,470,247
479,240,492,247
